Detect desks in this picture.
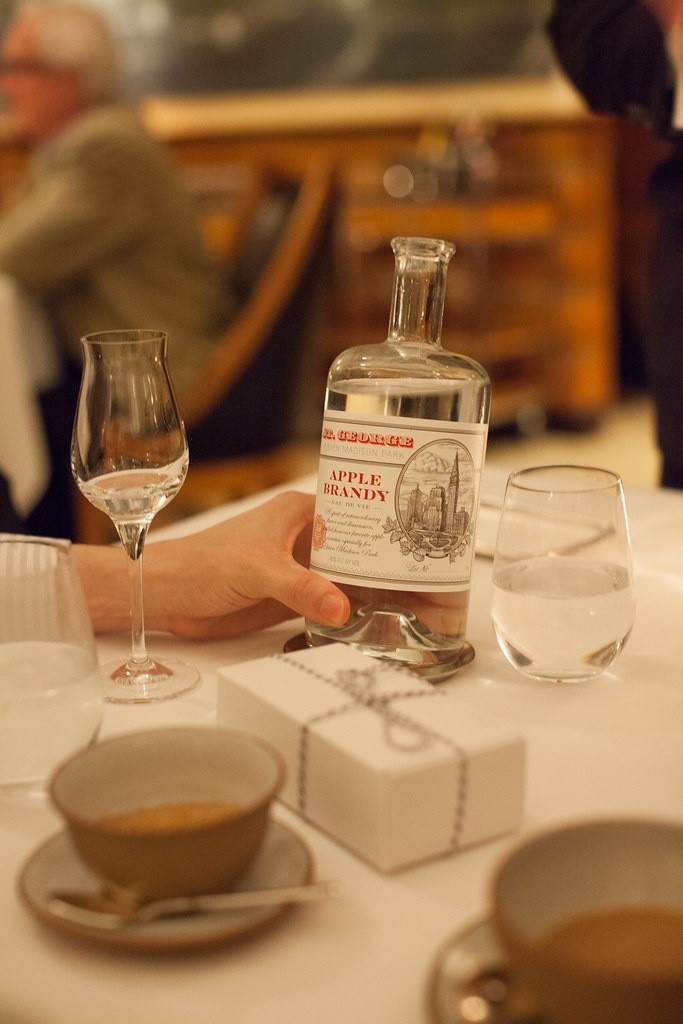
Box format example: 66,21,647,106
0,457,683,1024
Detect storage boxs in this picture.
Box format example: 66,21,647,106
214,643,527,873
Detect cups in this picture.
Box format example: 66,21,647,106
1,534,106,785
47,725,287,905
489,811,683,1024
488,463,636,684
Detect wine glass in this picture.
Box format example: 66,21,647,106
69,330,202,703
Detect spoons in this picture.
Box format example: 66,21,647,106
49,882,320,929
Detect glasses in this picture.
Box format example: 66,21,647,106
0,54,67,73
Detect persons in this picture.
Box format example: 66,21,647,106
0,0,228,546
0,490,466,644
542,0,683,492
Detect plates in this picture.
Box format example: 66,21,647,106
16,820,313,954
424,915,499,1024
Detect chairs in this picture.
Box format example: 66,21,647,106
53,145,335,544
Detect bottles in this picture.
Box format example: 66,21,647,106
304,234,493,670
